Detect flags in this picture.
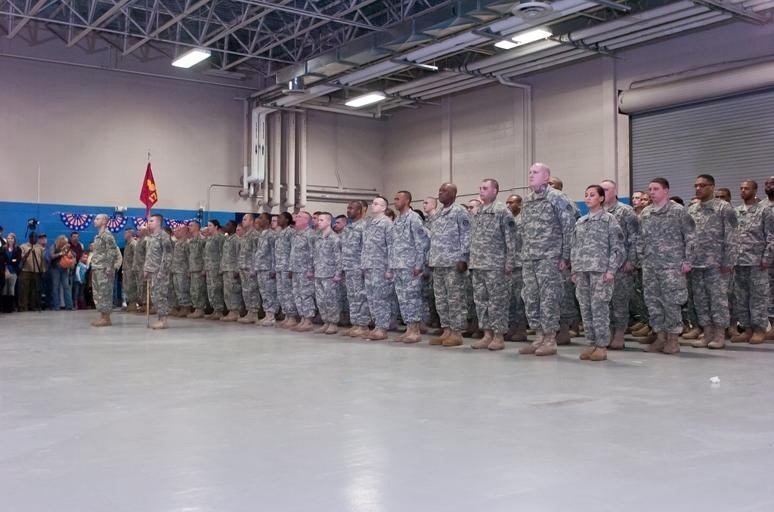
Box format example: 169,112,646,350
140,163,158,208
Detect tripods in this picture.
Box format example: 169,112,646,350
18,246,53,312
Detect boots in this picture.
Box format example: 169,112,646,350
8,296,14,310
95,312,112,326
559,316,774,363
394,325,557,356
275,315,388,340
187,308,276,326
91,312,103,325
80,297,87,309
1,295,8,313
125,302,190,317
148,316,168,329
74,298,79,310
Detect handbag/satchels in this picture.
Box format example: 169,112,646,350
59,255,74,269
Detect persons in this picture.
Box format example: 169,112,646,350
164,211,349,333
0,227,96,312
91,214,122,325
334,163,582,355
121,212,173,328
571,174,774,360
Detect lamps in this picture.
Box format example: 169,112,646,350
493,26,553,50
344,90,386,108
171,48,212,69
512,0,552,18
